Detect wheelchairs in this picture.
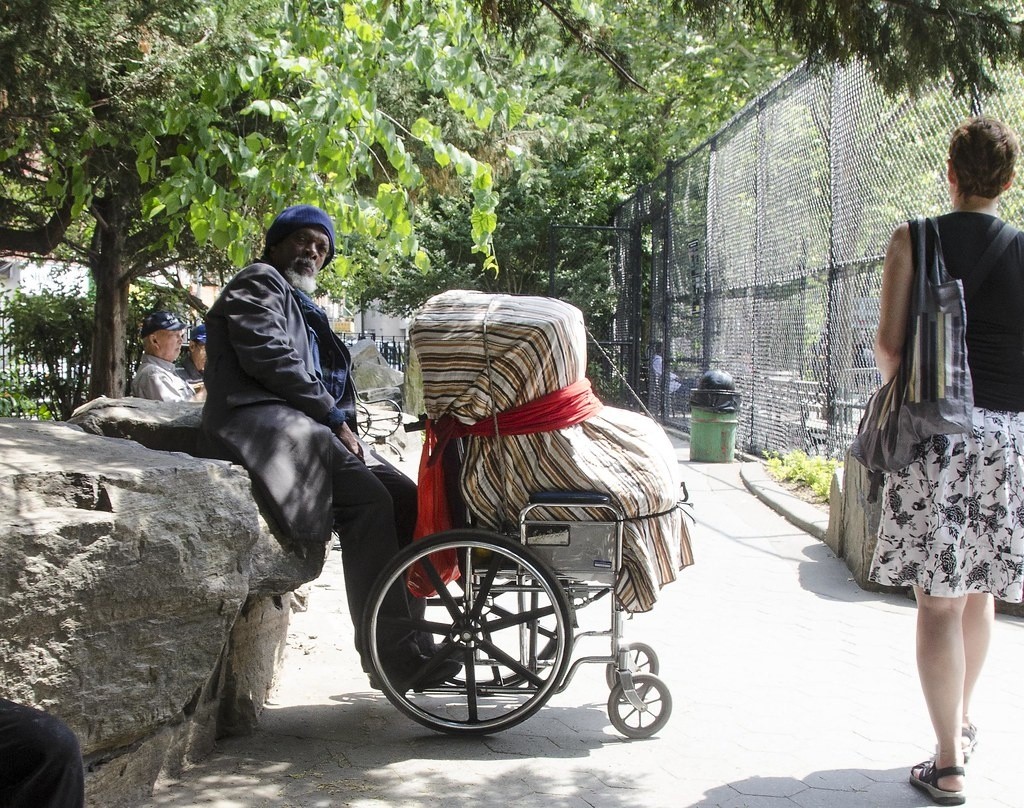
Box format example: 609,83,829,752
359,412,674,739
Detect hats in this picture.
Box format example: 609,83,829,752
264,205,335,271
191,324,207,343
141,312,191,339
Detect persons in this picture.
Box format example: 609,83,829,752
653,342,692,399
175,325,207,382
131,310,209,403
202,205,469,691
868,116,1024,806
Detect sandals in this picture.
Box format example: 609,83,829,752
961,723,978,763
909,759,967,804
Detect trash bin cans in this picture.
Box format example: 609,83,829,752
688,368,743,463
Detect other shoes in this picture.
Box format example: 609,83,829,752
434,643,465,662
366,655,463,690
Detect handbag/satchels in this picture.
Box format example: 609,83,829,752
405,419,461,598
849,215,973,474
